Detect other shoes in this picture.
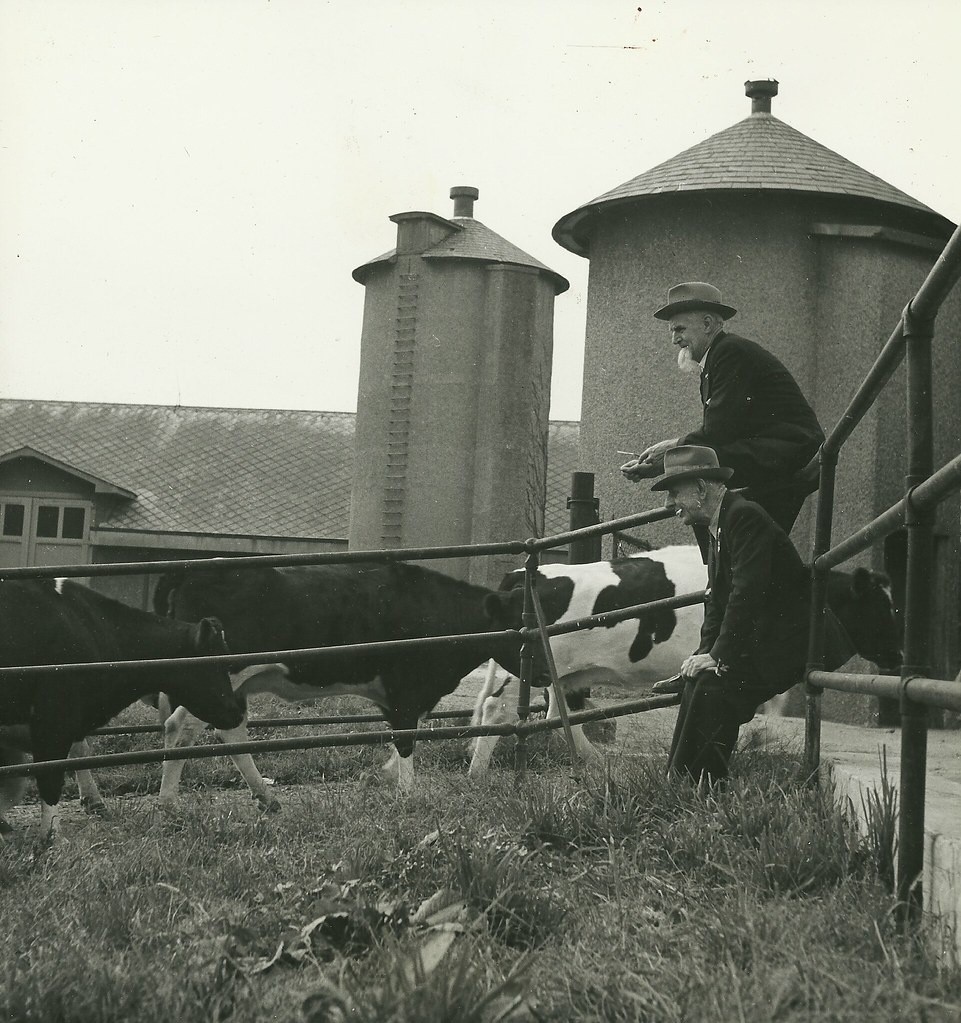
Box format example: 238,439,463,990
650,672,687,694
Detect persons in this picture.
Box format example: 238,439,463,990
647,443,858,799
617,281,827,594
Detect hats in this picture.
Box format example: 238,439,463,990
653,281,737,321
649,445,734,492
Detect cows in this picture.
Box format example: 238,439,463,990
0,577,247,849
153,557,558,816
465,543,906,781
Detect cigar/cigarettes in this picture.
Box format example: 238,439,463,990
617,449,641,459
675,508,682,516
